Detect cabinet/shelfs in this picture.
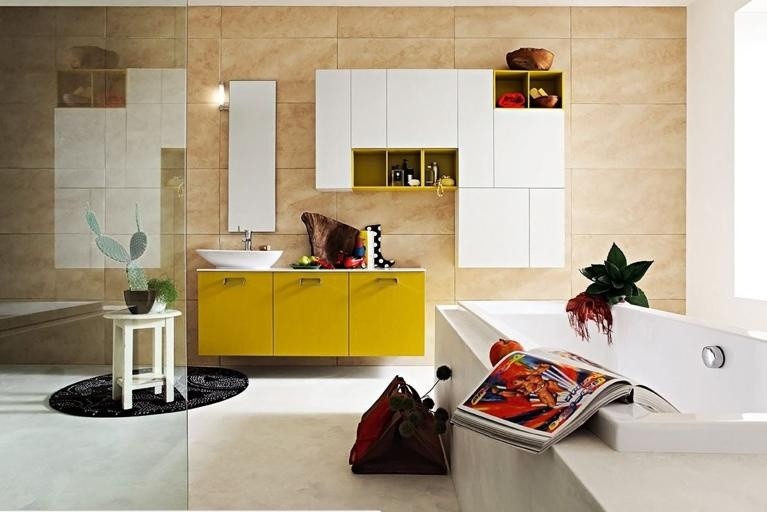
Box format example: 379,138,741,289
104,108,127,189
493,70,565,110
273,273,350,357
388,69,458,148
455,187,529,270
127,67,163,105
493,110,566,187
351,149,458,190
316,68,351,193
128,107,161,188
349,270,426,357
197,270,274,357
56,68,126,109
55,108,105,189
229,80,276,232
54,187,106,268
351,68,388,147
459,68,493,188
162,68,187,149
108,188,162,268
529,187,567,269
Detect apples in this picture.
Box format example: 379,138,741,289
489,338,523,367
298,255,316,266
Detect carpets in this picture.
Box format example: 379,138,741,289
48,364,249,419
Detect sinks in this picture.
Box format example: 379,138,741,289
196,249,283,269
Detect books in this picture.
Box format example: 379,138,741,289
355,229,376,270
448,343,682,454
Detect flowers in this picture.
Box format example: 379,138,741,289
566,243,654,348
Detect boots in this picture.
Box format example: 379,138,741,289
366,224,395,268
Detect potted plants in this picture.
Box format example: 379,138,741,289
81,200,183,314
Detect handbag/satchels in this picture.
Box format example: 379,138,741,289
348,375,448,474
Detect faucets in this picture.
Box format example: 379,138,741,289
241,229,252,250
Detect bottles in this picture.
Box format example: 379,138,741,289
426,161,439,186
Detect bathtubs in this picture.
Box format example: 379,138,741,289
455,299,766,454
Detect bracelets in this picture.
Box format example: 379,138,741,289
495,389,503,395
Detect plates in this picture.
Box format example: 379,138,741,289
290,264,321,269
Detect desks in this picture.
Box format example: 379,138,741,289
105,309,182,413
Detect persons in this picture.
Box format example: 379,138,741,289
490,361,567,408
